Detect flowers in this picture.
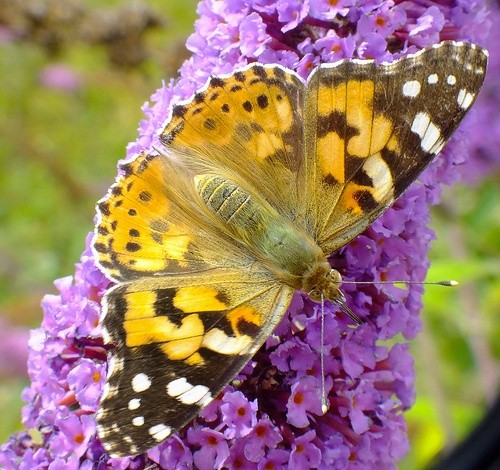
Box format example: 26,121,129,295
1,0,500,470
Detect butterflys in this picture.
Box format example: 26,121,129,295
90,39,489,460
